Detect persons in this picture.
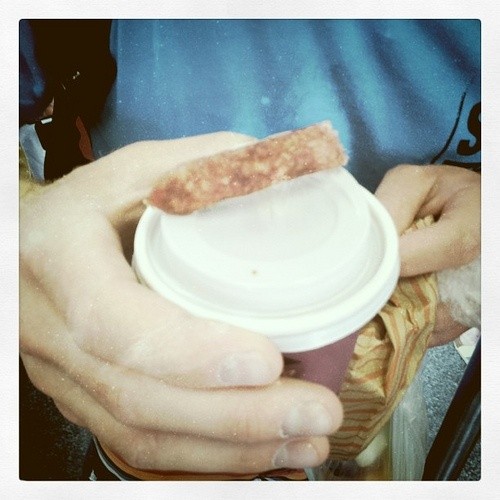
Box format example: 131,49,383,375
20,18,481,481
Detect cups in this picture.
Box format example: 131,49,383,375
128,165,401,397
